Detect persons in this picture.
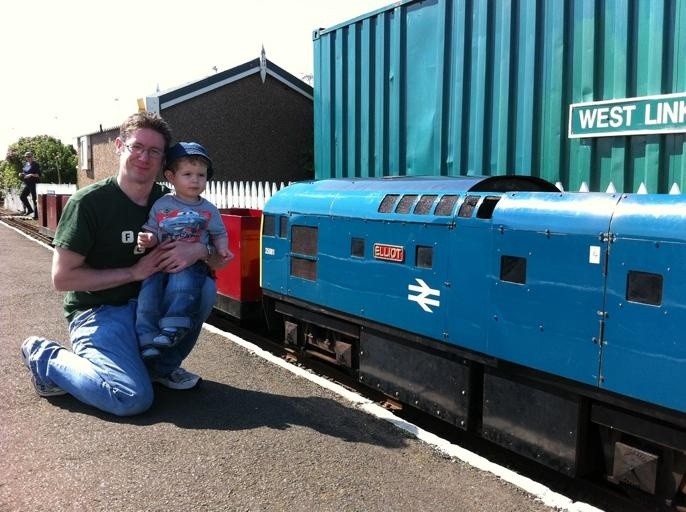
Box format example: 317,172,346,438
135,143,235,360
18,111,230,418
18,152,40,219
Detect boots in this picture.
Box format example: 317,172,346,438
24,207,37,218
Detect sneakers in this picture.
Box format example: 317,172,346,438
140,327,185,359
20,336,68,397
152,367,203,390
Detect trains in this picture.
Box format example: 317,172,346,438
35,174,685,510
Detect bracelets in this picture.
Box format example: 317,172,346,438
205,243,213,263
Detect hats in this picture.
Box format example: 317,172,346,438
163,142,214,181
22,152,32,157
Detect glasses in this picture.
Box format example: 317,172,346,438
123,142,161,159
